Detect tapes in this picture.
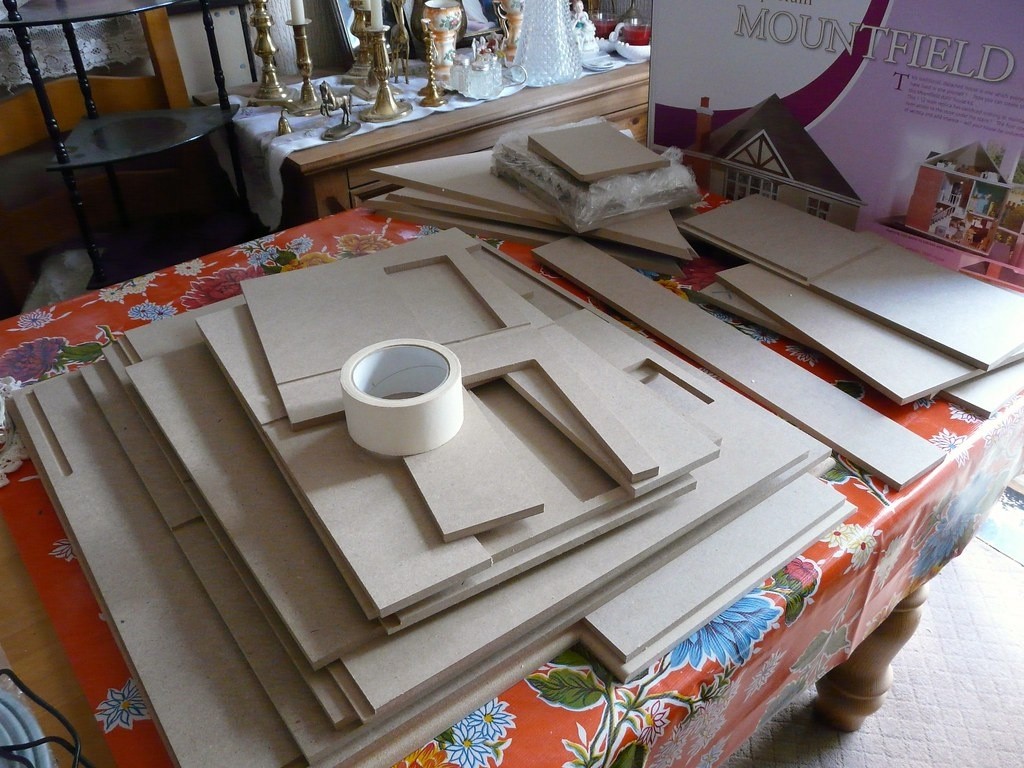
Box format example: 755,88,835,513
340,339,465,457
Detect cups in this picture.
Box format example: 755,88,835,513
590,13,619,39
623,16,651,46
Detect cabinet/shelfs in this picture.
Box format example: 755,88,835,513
1,0,258,285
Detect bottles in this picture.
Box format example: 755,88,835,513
450,59,472,92
469,62,494,97
480,54,502,87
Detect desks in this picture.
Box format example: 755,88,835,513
0,185,1024,768
193,50,649,219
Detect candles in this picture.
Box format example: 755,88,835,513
289,0,305,23
363,0,370,8
369,0,383,28
